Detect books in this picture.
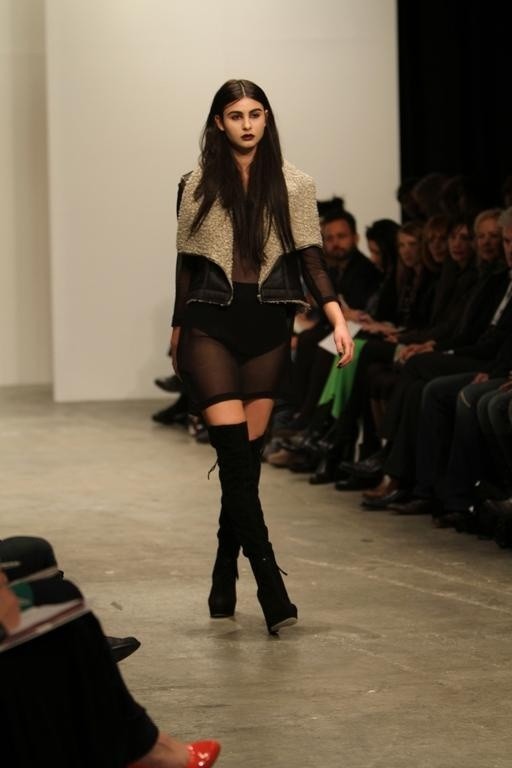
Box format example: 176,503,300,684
1,597,94,657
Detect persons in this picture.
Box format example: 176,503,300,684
0,534,143,665
165,78,355,639
1,573,225,768
151,374,208,443
263,197,512,553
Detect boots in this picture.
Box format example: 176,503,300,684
207,420,297,632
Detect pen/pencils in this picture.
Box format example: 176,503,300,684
0,561,21,569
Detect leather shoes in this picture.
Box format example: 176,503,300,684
267,434,507,550
104,636,142,663
152,406,182,424
184,740,221,768
154,374,181,392
